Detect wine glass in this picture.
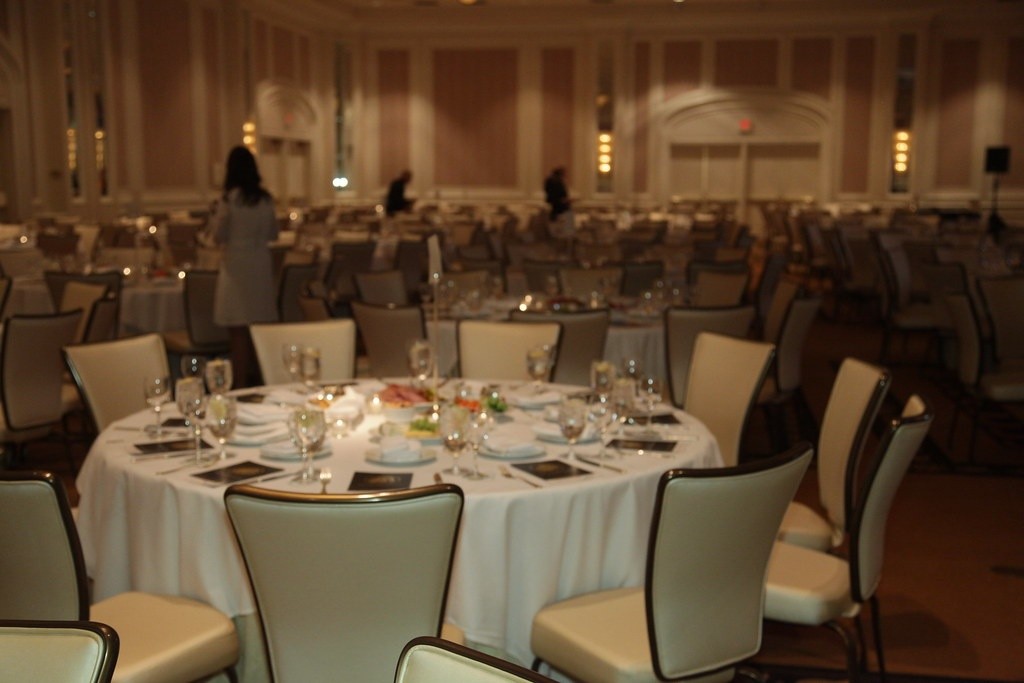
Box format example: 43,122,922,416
558,399,586,462
206,393,237,463
184,393,211,467
280,345,321,395
143,374,171,438
287,409,326,485
592,349,661,425
526,344,552,395
441,410,490,482
408,341,433,390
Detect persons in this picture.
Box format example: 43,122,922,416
542,166,571,222
203,145,279,393
384,168,418,219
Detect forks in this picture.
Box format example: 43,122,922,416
318,467,332,493
500,466,542,488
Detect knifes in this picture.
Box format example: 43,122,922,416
434,473,443,484
582,460,621,472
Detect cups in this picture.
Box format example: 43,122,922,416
176,355,232,424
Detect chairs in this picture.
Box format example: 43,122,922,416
248,317,357,385
456,318,564,382
778,356,896,552
394,636,560,683
760,393,932,682
0,619,120,683
0,467,243,683
530,439,813,683
224,483,464,683
58,331,174,434
0,193,1021,473
683,329,777,466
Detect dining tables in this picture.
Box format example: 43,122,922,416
74,377,723,683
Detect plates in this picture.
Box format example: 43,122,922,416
512,392,568,408
475,439,547,458
259,443,333,461
536,426,604,444
365,449,437,467
221,390,290,446
546,406,564,422
